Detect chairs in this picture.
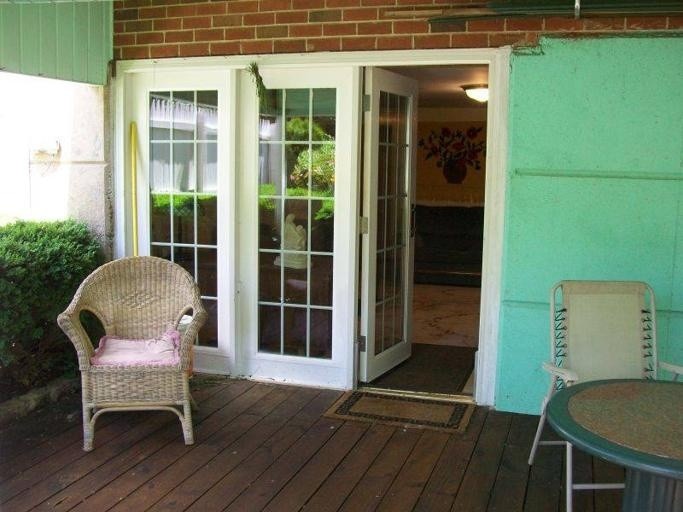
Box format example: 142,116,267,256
54,254,210,455
523,278,682,512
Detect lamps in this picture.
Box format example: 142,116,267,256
459,83,488,102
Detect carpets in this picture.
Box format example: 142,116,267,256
320,339,485,439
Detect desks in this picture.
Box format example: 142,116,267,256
544,375,683,512
